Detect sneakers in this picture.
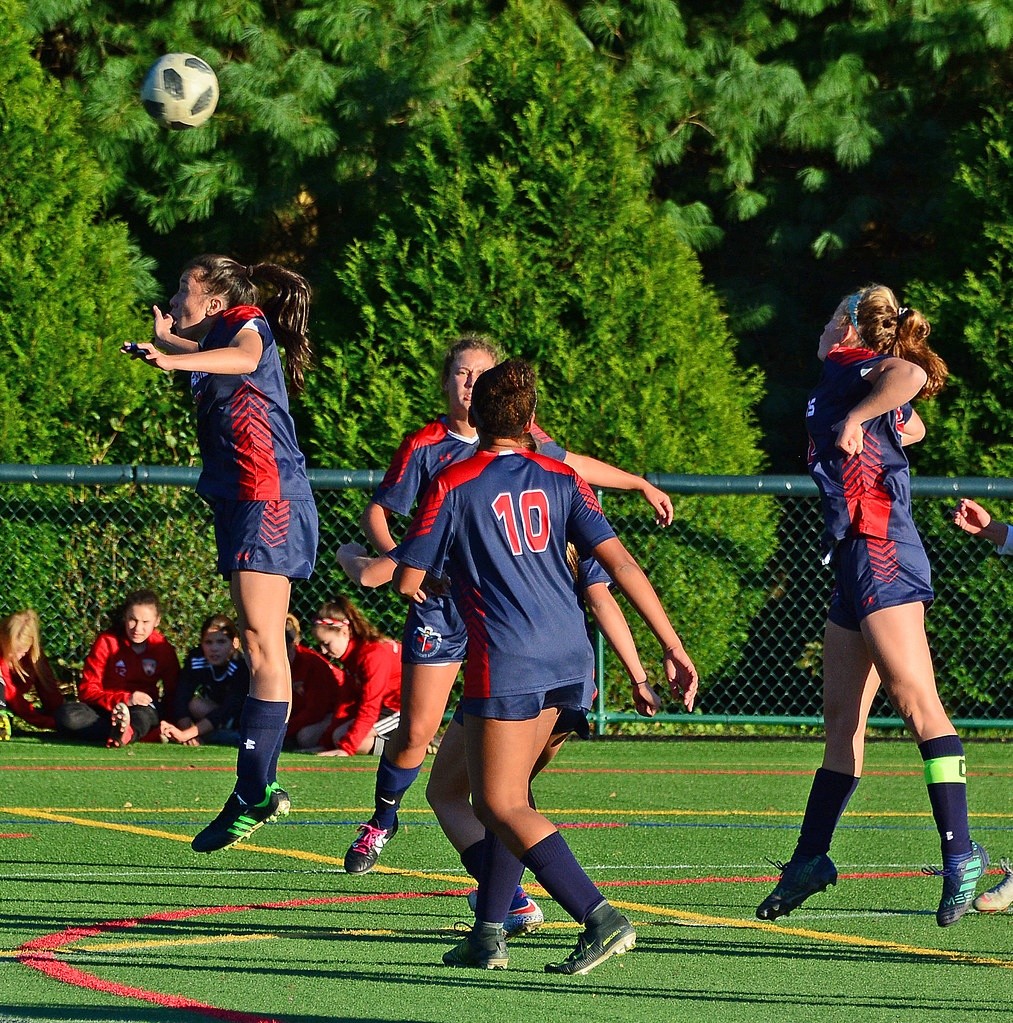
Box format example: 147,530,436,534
343,814,399,877
440,922,509,969
190,782,291,852
921,840,989,927
545,910,636,978
972,857,1013,913
469,890,543,937
755,854,837,922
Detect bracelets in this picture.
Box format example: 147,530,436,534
631,678,648,685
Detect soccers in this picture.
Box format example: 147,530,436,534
139,51,220,128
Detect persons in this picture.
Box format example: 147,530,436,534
345,336,674,874
297,596,407,759
337,532,666,938
283,613,346,754
118,254,321,853
756,287,986,927
0,607,67,743
953,495,1013,913
390,361,702,974
54,589,183,750
160,613,248,748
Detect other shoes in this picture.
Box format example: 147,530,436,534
106,702,131,748
